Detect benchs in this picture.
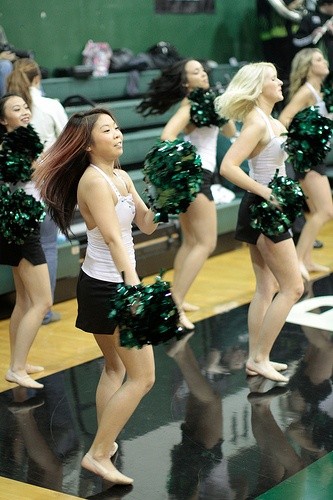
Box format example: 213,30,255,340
0,61,302,294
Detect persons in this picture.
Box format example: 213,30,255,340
7,57,69,152
278,48,332,281
229,373,302,492
9,390,63,495
293,0,333,48
0,95,53,390
132,58,237,330
296,281,333,455
81,446,134,500
214,63,305,384
258,0,299,106
30,108,205,485
0,43,14,97
169,331,235,500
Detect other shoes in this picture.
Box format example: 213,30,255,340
6,370,44,389
50,310,63,324
80,453,134,484
109,441,119,458
26,365,45,373
245,360,287,376
246,360,289,382
